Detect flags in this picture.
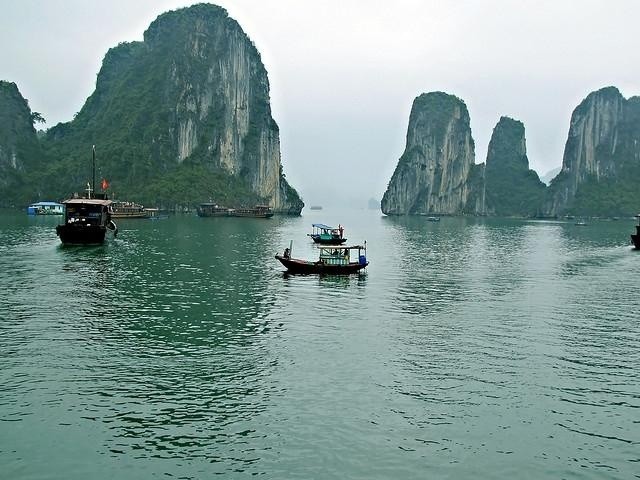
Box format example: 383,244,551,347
102,177,108,191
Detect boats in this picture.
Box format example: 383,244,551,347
309,205,323,210
195,203,233,217
427,216,441,222
630,213,640,251
108,192,148,218
575,221,587,227
51,143,119,244
306,223,347,246
232,203,274,218
273,238,371,276
